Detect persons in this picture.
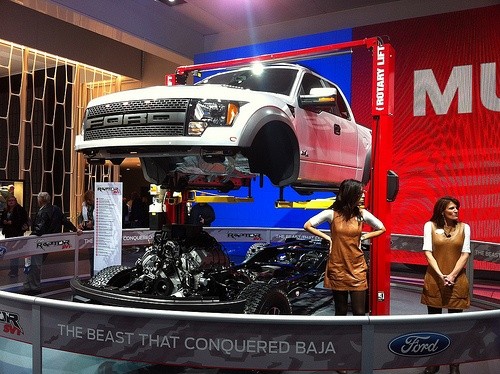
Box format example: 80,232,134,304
79,190,93,277
419,196,472,374
24,192,85,289
304,179,386,316
191,193,216,227
123,192,149,253
3,196,31,277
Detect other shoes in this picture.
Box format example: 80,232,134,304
425,365,440,374
8,272,18,277
449,364,460,374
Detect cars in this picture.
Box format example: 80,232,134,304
75,62,372,194
